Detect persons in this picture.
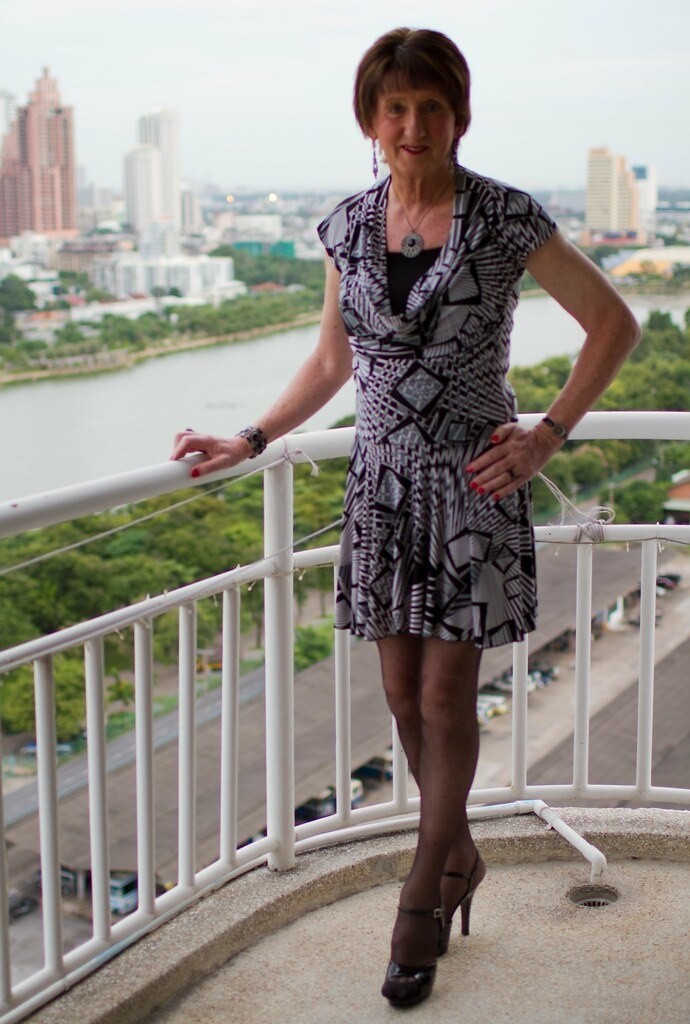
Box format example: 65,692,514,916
169,27,639,1008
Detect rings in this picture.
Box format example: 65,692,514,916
507,468,516,481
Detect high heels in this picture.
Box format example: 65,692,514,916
382,894,446,1008
438,850,487,956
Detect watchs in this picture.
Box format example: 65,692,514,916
537,417,571,443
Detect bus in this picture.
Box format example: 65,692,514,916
109,874,139,914
363,751,393,780
295,779,363,820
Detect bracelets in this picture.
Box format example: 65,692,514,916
234,426,266,458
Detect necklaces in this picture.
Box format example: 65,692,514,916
390,171,457,258
